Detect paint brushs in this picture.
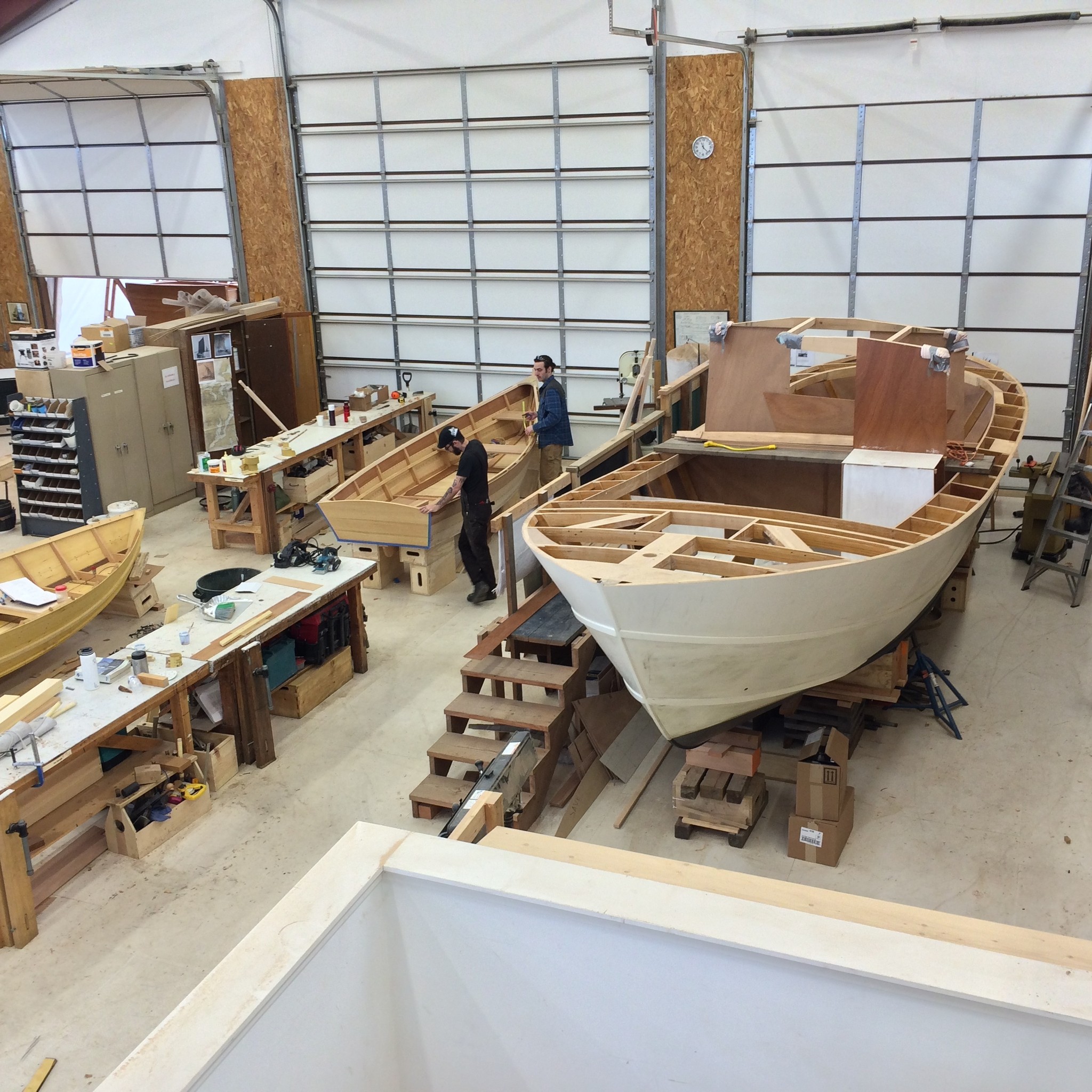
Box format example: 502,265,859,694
181,622,194,645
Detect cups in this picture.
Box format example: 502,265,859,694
9,400,24,412
23,464,31,471
347,416,355,425
316,415,324,426
129,675,144,692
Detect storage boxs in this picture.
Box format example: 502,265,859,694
787,728,855,867
125,314,148,349
282,458,339,502
80,318,130,352
8,328,59,370
341,430,396,470
71,341,106,368
349,383,388,411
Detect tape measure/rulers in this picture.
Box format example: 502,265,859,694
279,428,307,443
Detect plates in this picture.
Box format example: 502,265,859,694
158,670,177,681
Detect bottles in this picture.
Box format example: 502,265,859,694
131,650,149,675
343,402,350,422
55,584,68,603
221,451,232,475
72,335,87,343
328,404,336,426
77,646,100,691
30,402,47,413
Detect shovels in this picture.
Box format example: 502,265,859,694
177,593,254,624
402,372,418,433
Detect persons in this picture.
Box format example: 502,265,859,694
418,425,499,604
522,355,574,485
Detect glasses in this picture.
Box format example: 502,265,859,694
445,442,453,450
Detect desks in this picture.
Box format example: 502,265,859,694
0,549,379,951
186,392,436,554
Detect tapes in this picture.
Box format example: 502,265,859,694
244,455,259,465
281,447,296,457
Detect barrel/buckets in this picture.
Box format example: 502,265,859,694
86,514,113,524
207,460,220,473
0,499,16,531
179,631,190,645
46,351,68,369
107,500,138,517
192,567,263,603
198,451,211,472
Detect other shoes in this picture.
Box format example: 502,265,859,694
467,582,498,603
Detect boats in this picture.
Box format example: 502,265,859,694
521,315,1030,750
0,507,147,677
315,369,539,549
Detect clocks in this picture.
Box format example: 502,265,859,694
692,136,714,159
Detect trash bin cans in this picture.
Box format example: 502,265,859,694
193,567,261,603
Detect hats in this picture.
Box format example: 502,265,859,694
437,426,459,448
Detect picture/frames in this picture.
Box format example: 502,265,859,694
6,302,31,324
674,311,729,349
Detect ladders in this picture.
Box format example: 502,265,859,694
1020,400,1092,609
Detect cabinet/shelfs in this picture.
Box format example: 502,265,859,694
5,302,321,539
